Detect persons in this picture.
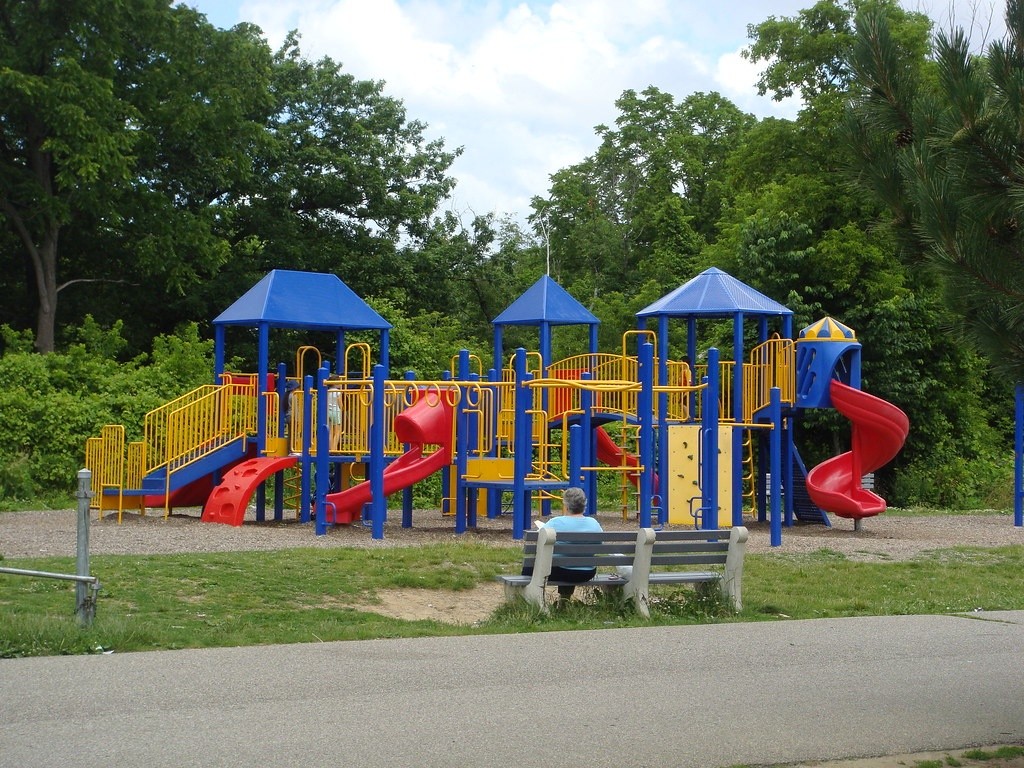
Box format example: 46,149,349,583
310,464,338,503
284,380,301,453
520,487,604,602
678,356,692,423
326,375,343,456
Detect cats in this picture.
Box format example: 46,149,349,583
608,554,633,580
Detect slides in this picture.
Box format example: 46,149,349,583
805,379,908,516
593,426,659,507
315,389,455,525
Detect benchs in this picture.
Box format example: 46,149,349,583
494,526,749,622
764,473,874,504
